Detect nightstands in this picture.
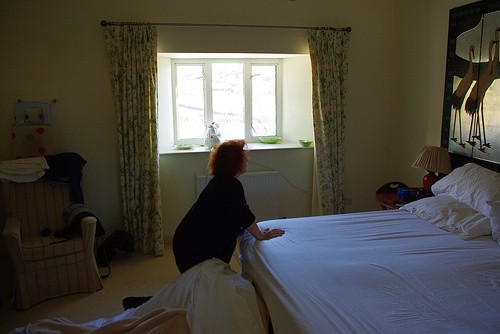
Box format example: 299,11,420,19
374,182,426,210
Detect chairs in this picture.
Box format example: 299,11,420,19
0,152,105,311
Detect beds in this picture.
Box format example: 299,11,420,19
239,209,500,334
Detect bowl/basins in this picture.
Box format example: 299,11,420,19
299,139,313,147
258,136,282,144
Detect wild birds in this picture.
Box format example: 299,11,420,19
205,122,221,153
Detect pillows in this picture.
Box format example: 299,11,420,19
399,195,492,241
431,163,500,244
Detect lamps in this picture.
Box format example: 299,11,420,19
410,146,453,195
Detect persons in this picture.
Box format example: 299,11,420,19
121,140,285,311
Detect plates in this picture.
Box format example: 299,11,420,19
174,143,194,149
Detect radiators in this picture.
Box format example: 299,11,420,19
196,171,279,220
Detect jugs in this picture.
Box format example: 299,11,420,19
205,125,221,149
